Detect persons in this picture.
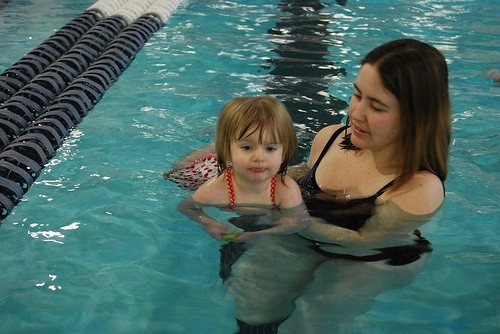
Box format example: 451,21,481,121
161,95,312,243
217,37,453,334
